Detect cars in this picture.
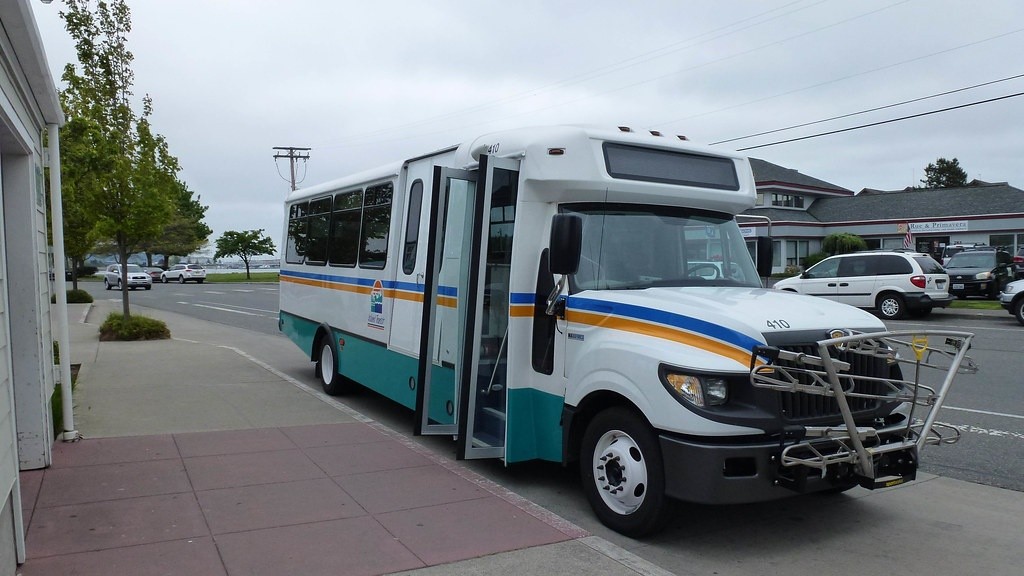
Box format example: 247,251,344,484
1013,246,1024,267
998,278,1024,327
770,250,954,321
103,263,152,291
942,243,988,267
142,267,164,282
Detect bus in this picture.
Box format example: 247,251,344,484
277,125,975,537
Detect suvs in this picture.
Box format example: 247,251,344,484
942,248,1017,300
159,263,206,284
